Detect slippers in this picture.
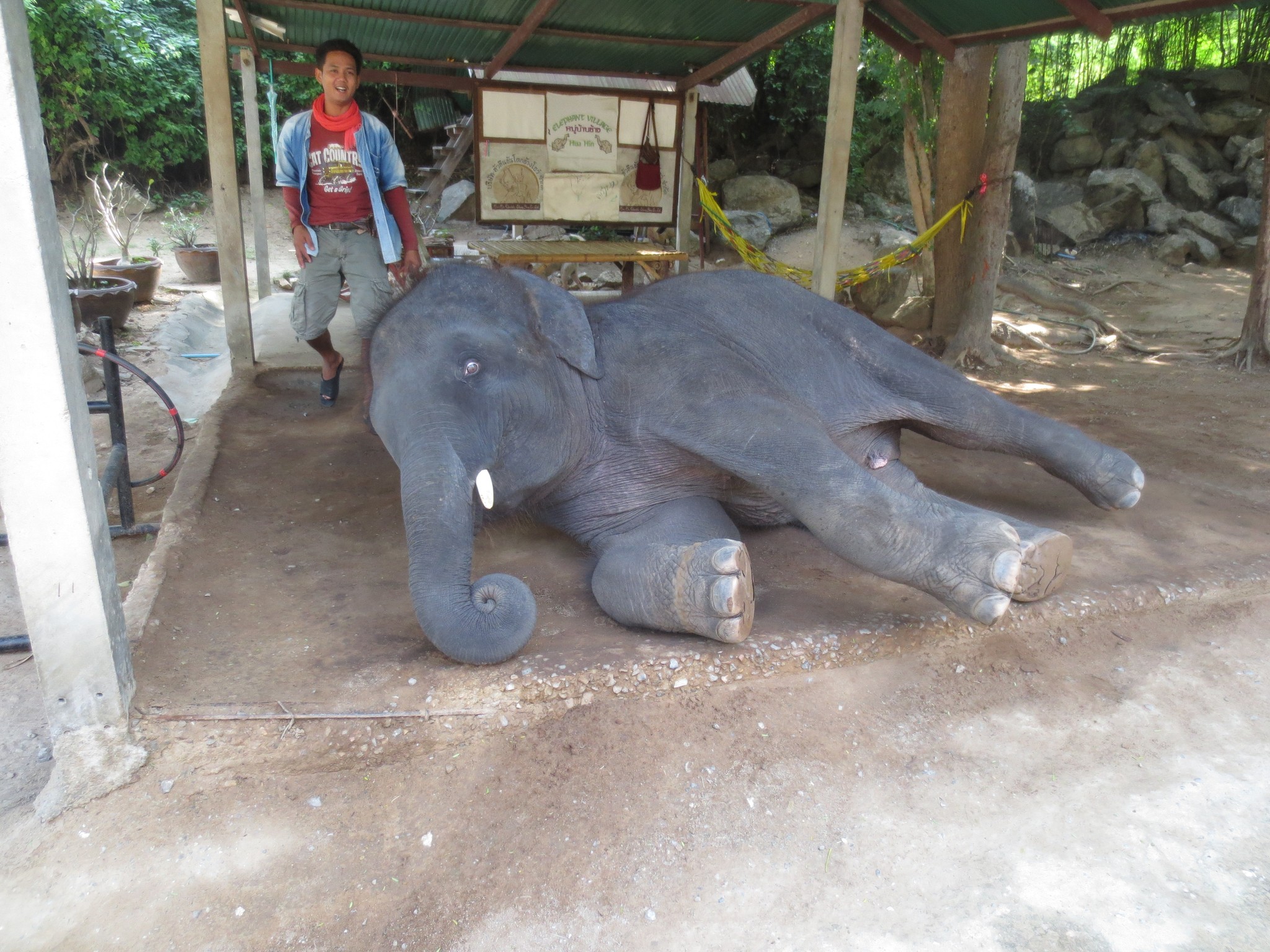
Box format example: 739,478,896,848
364,415,378,435
320,358,343,405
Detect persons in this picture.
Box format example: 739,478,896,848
275,38,422,408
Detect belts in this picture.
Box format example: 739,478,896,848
322,220,370,230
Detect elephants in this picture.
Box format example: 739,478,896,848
370,264,1145,666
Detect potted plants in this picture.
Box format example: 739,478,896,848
56,197,138,334
86,163,163,305
407,192,456,284
163,192,223,283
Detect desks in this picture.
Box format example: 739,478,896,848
467,240,688,297
